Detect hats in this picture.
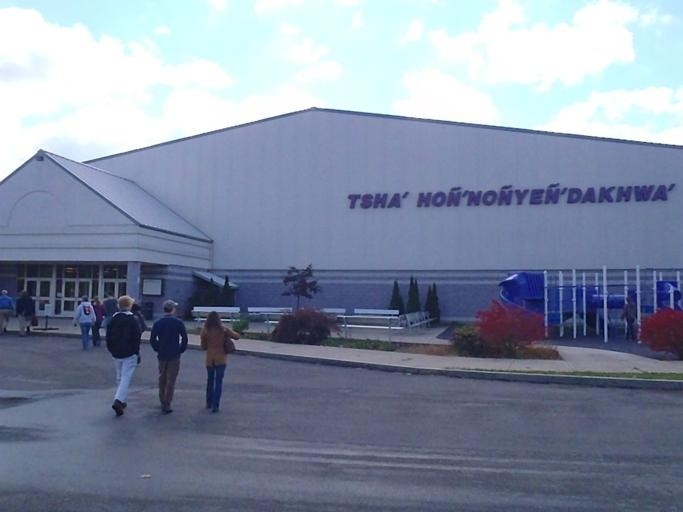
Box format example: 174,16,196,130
119,296,134,306
164,300,178,309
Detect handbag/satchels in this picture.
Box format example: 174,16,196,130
223,327,234,353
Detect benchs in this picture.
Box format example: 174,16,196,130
190,306,436,344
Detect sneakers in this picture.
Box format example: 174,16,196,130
112,400,126,416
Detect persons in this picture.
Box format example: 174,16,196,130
0,288,15,335
149,299,188,413
104,295,142,415
200,310,240,413
17,290,34,336
618,295,637,342
72,291,146,363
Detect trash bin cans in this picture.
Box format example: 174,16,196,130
141,301,153,320
